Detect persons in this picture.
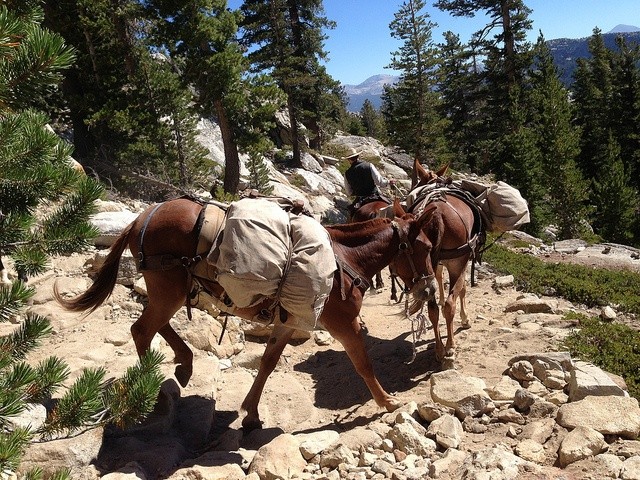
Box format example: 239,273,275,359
341,147,394,201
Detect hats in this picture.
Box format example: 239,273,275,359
341,148,363,159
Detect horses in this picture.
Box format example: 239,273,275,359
350,198,390,295
48,197,440,434
407,157,478,363
